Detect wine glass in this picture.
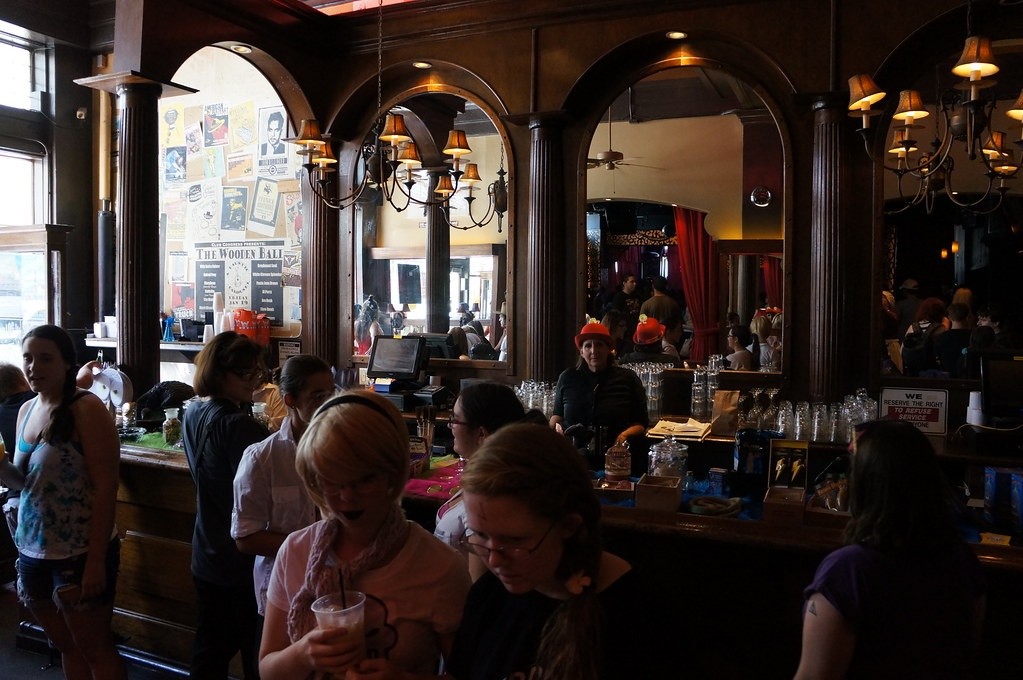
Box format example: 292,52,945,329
737,387,780,432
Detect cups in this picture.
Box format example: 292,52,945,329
202,292,236,345
621,352,725,420
965,391,984,433
310,591,368,666
507,380,560,420
780,387,878,443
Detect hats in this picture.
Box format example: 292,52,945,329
494,301,506,317
632,315,665,346
574,313,617,350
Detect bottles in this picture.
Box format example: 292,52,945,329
646,433,688,479
162,407,183,447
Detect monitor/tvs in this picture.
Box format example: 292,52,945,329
407,333,459,358
366,335,426,380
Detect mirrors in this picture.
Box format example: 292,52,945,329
585,67,784,373
354,92,508,361
882,37,1023,373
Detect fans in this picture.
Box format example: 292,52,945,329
588,106,668,171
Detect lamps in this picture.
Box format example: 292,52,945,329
888,103,1017,217
847,0,1023,216
433,140,508,233
293,0,471,213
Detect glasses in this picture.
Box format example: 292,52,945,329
239,372,265,385
458,523,555,564
446,413,475,427
312,469,386,497
727,333,734,337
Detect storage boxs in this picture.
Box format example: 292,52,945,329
763,439,809,527
635,473,682,512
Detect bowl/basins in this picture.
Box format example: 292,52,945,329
117,427,147,444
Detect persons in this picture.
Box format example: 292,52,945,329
355,298,384,355
258,389,472,680
434,383,548,585
724,316,782,371
792,418,987,680
181,331,288,680
0,325,121,680
444,421,684,680
612,273,683,366
549,322,648,471
448,302,507,362
882,279,1013,378
230,355,336,617
